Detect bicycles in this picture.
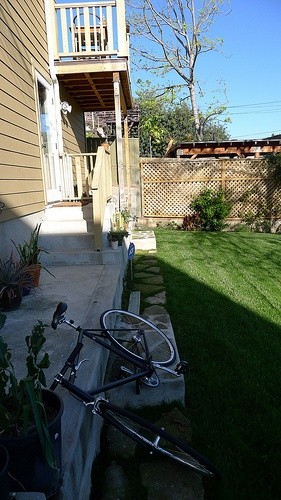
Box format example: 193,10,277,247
45,298,219,483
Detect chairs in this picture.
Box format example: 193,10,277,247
73,12,111,60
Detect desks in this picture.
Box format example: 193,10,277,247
69,25,107,59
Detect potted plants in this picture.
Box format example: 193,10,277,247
110,210,132,250
0,224,54,311
0,312,63,500
